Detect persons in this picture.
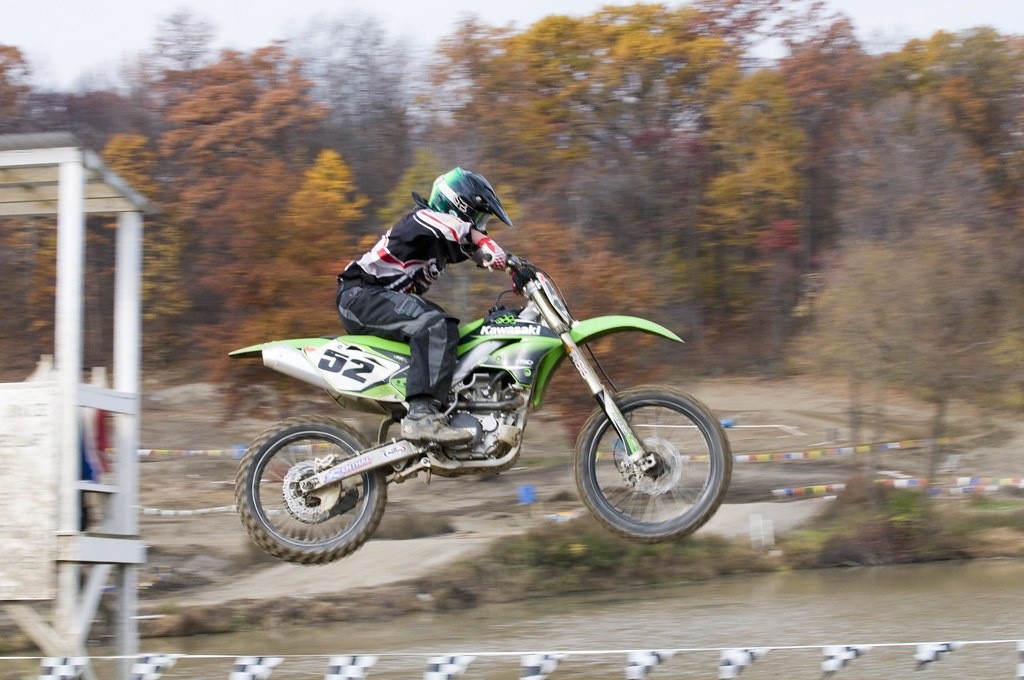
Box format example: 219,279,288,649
334,166,513,444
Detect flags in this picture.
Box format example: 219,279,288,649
80,408,111,480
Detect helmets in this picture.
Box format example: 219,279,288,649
428,167,513,232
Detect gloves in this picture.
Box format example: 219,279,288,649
506,264,525,296
478,237,507,273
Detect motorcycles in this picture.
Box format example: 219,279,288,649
225,253,733,564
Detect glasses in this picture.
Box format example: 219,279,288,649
473,211,492,230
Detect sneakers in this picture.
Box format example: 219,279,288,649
401,397,473,446
